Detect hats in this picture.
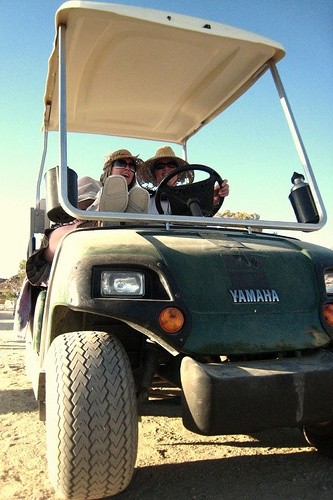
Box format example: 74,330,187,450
103,150,144,170
137,146,195,191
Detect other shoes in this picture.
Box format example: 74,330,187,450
100,174,128,227
122,187,150,225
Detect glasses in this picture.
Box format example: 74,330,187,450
155,162,178,169
113,159,140,173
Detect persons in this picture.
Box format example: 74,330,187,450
136,146,229,215
25,150,150,285
